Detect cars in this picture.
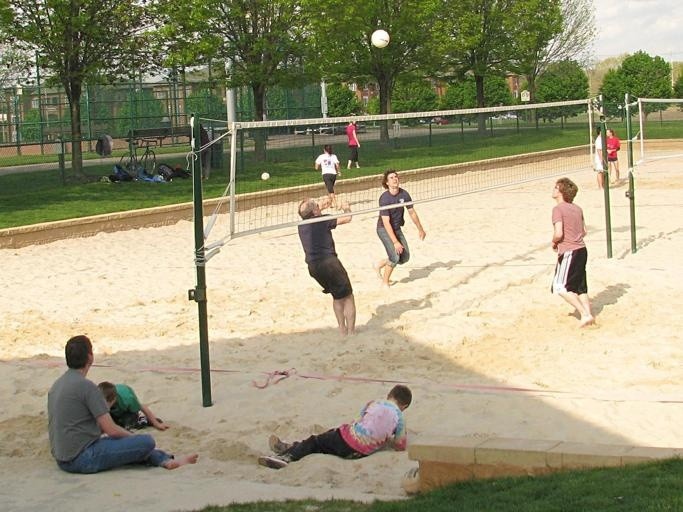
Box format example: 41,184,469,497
419,117,448,126
503,111,522,120
488,115,507,121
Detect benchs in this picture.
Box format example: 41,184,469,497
166,123,191,145
124,127,168,149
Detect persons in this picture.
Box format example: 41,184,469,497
296,198,357,337
258,384,413,470
606,128,624,185
371,168,427,289
549,176,595,330
46,334,200,475
345,119,361,170
97,381,171,431
314,144,342,209
199,122,212,180
593,126,605,192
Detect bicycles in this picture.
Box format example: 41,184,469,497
117,137,157,175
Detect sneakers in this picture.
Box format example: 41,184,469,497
268,435,294,454
258,455,291,469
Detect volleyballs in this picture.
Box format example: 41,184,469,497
261,173,269,180
372,30,390,49
